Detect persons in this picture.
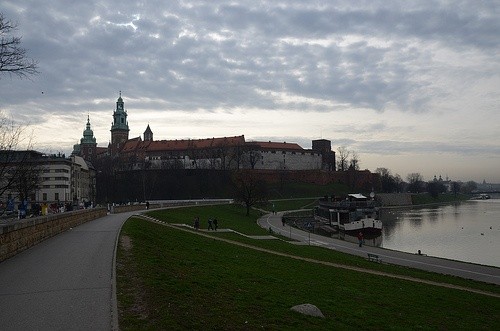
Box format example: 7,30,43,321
273,209,275,215
146,201,149,210
47,202,73,215
195,217,199,231
358,232,363,247
208,217,214,230
80,200,105,208
213,217,218,230
113,199,126,206
282,217,286,226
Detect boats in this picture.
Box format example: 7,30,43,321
315,192,386,237
